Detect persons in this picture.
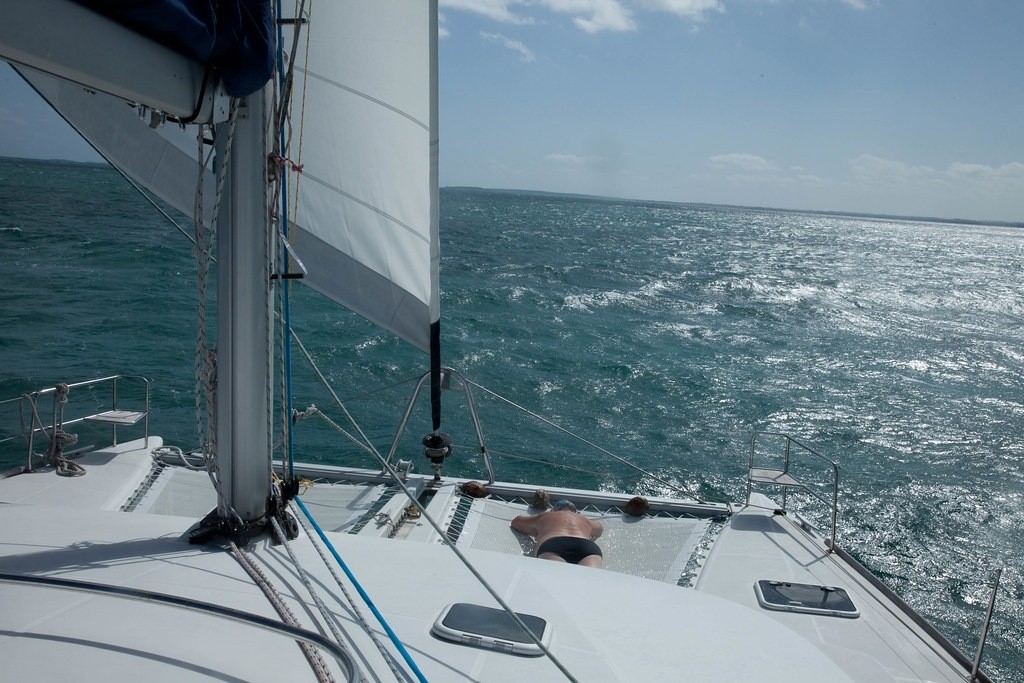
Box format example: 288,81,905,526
511,500,604,568
